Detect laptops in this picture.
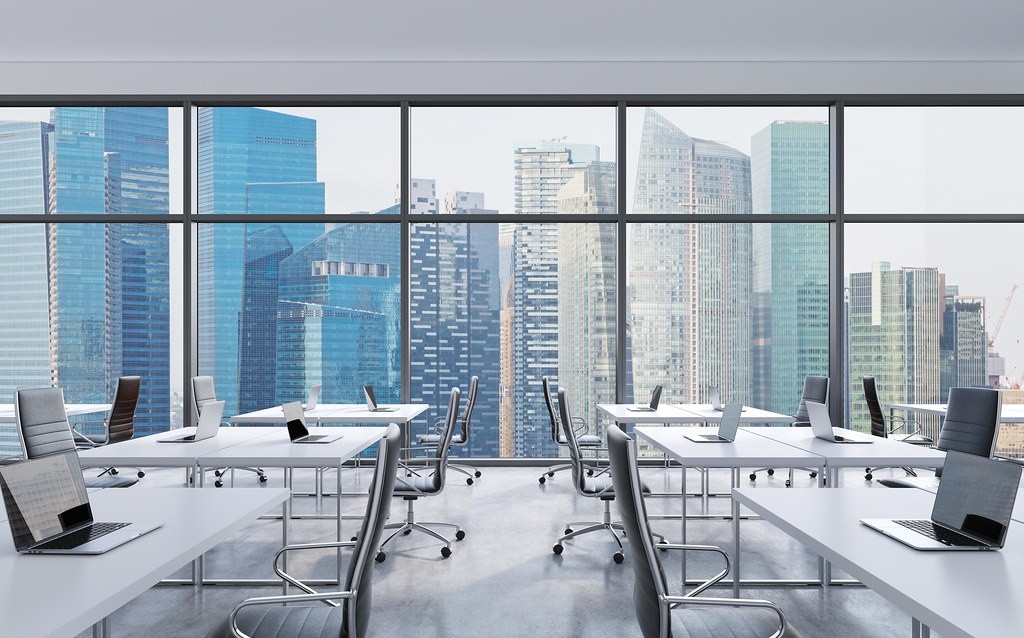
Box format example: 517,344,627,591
860,450,1023,550
282,401,344,443
281,385,320,412
684,401,744,443
805,401,873,443
363,385,399,412
157,401,225,443
0,450,165,555
627,385,662,412
708,385,746,412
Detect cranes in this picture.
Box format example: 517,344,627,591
987,284,1018,347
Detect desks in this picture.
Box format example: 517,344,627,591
594,401,1023,638
1,404,430,638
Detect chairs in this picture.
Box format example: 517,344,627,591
205,423,402,638
16,387,139,488
748,376,831,488
539,376,613,484
408,376,481,486
372,386,465,562
189,376,268,486
72,376,146,480
877,387,1002,489
605,424,786,638
552,387,670,563
861,375,933,480
